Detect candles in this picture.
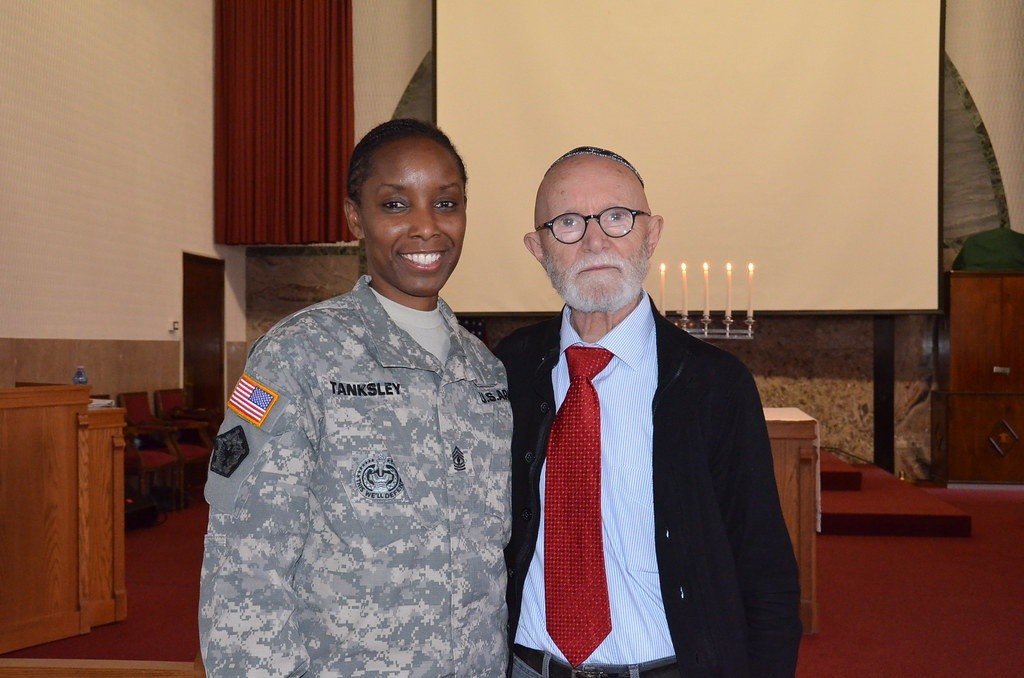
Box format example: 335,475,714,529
660,264,665,317
703,262,710,316
747,263,754,318
681,263,689,316
726,263,732,317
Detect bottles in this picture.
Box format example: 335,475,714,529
73,366,87,385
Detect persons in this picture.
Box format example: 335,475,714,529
492,147,802,678
197,119,513,678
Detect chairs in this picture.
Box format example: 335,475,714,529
91,387,224,533
